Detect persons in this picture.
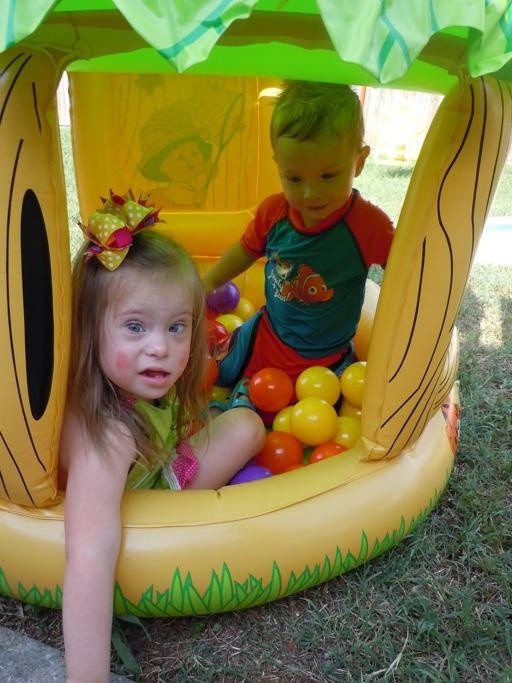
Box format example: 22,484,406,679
200,79,396,427
57,226,270,683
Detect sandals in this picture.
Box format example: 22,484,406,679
200,401,228,415
228,378,257,410
